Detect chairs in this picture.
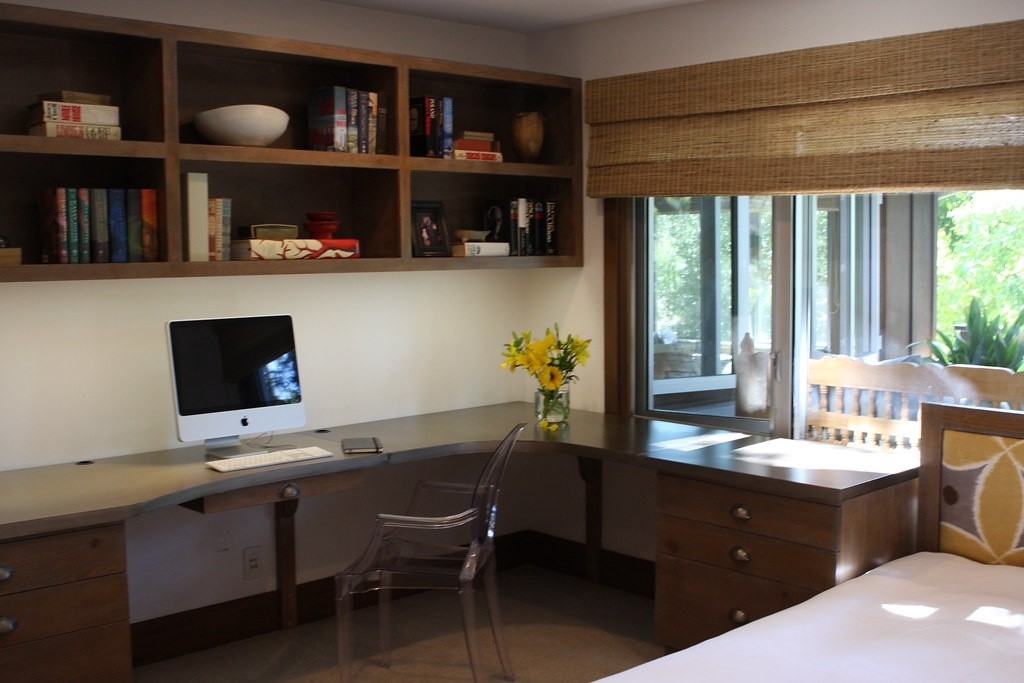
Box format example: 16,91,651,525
335,422,527,683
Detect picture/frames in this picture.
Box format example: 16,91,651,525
411,200,453,258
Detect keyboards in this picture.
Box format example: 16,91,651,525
205,446,333,473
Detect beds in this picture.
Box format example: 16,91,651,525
590,399,1024,683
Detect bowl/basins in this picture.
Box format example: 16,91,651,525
195,104,289,146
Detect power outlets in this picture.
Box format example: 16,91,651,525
243,545,264,581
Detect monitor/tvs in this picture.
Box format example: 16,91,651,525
166,315,305,459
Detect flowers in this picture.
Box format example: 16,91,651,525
499,321,592,390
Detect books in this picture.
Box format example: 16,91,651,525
40,187,158,264
410,94,503,163
449,198,557,258
182,172,361,261
308,86,379,154
26,91,122,140
341,437,384,454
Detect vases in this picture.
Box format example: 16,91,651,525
534,391,569,431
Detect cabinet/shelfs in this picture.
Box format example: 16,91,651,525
0,520,133,683
0,3,584,282
651,471,918,655
